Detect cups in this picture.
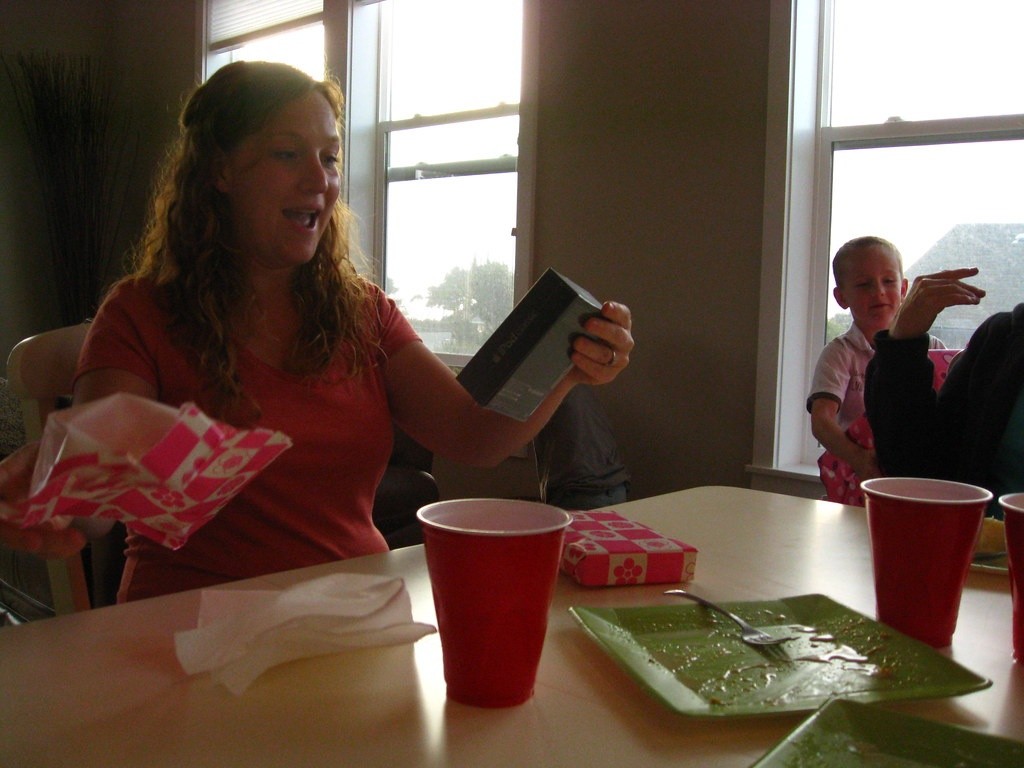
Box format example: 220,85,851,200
859,477,993,648
418,498,574,707
999,492,1024,665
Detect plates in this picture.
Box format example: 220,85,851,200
569,592,993,718
748,698,1024,768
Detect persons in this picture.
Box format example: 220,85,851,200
524,389,631,511
863,268,1024,520
0,61,634,606
806,235,946,483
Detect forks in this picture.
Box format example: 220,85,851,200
663,589,799,645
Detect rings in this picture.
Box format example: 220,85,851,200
605,351,616,367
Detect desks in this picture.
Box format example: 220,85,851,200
1,485,1024,768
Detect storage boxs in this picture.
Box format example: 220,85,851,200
817,349,969,507
456,267,605,423
561,510,701,588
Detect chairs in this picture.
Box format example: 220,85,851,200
8,320,128,617
